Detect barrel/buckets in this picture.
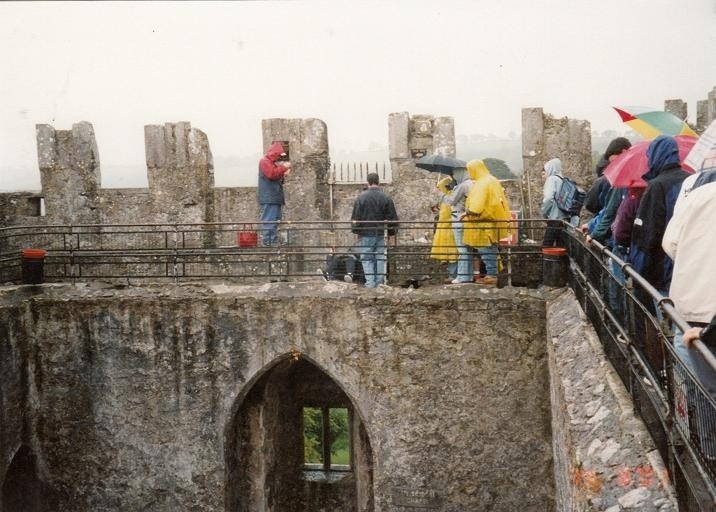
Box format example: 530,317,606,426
542,247,567,286
239,224,257,246
20,250,47,284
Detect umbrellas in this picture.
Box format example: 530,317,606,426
415,154,467,174
611,101,700,142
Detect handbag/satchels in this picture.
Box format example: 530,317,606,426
237,223,257,247
498,212,519,245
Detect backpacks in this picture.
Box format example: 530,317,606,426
554,175,587,215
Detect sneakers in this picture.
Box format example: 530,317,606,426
317,268,330,282
444,275,497,284
344,273,354,283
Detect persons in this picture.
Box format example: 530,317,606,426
583,116,715,463
539,157,572,247
349,171,399,288
257,141,289,246
458,157,516,284
432,168,474,284
431,175,458,282
